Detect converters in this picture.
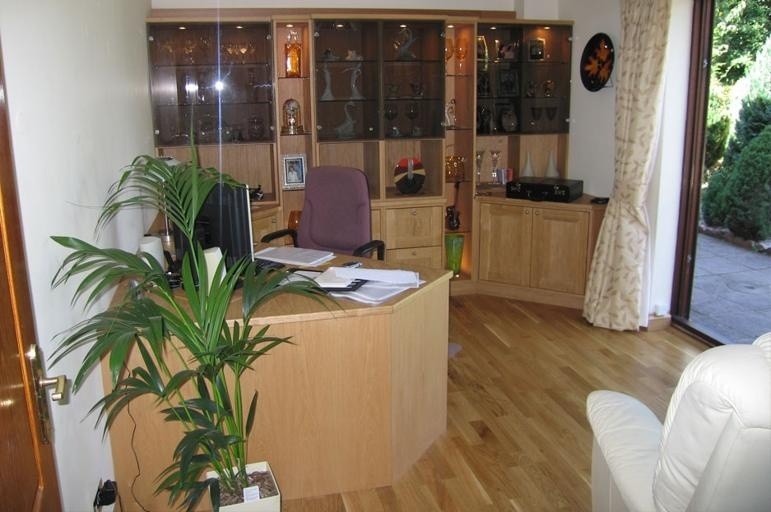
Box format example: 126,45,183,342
100,480,118,506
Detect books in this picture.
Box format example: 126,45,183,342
254,246,426,305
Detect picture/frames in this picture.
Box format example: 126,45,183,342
280,153,308,190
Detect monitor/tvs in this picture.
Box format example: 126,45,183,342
161,181,254,292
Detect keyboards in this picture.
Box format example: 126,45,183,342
252,256,282,279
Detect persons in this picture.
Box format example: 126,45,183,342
288,166,298,183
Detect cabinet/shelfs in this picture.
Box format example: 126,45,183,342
271,19,314,215
447,21,478,281
315,20,449,269
479,18,572,198
475,200,607,310
147,20,285,246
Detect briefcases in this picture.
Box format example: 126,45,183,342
506,176,583,202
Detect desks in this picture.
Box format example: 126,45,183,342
99,254,454,510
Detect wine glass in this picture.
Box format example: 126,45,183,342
444,38,466,76
530,106,557,132
488,149,500,186
474,150,483,189
386,103,418,138
149,34,257,64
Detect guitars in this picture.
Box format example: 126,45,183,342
446,182,460,231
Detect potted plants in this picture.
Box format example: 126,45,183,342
53,113,346,511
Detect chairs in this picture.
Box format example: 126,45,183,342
587,329,770,512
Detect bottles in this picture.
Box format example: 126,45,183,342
283,28,302,78
158,67,273,104
522,144,534,178
158,111,265,143
547,148,559,178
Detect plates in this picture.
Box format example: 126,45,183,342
394,158,425,195
581,31,616,92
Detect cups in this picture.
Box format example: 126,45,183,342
445,156,466,179
499,168,513,186
385,83,428,100
